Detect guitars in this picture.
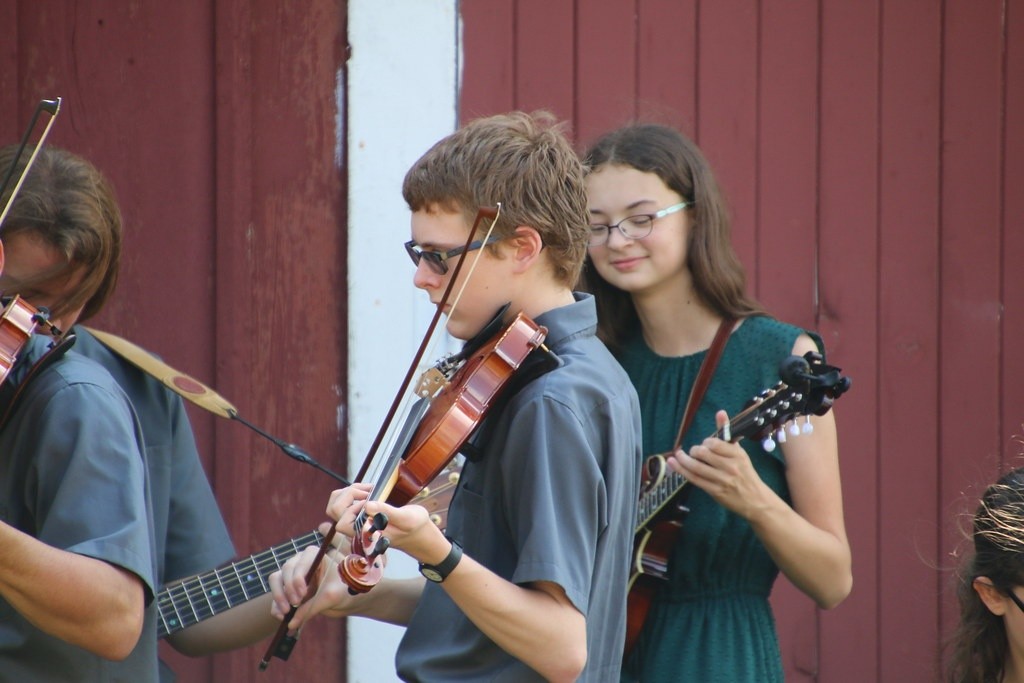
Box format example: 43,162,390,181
155,462,459,640
624,349,851,661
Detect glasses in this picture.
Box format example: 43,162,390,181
404,235,498,275
587,201,694,247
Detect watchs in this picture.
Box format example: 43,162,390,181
418,535,463,585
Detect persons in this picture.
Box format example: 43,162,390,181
949,466,1023,683
1,142,284,682
268,109,643,681
583,123,852,682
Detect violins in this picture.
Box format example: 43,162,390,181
338,300,550,596
0,294,48,386
